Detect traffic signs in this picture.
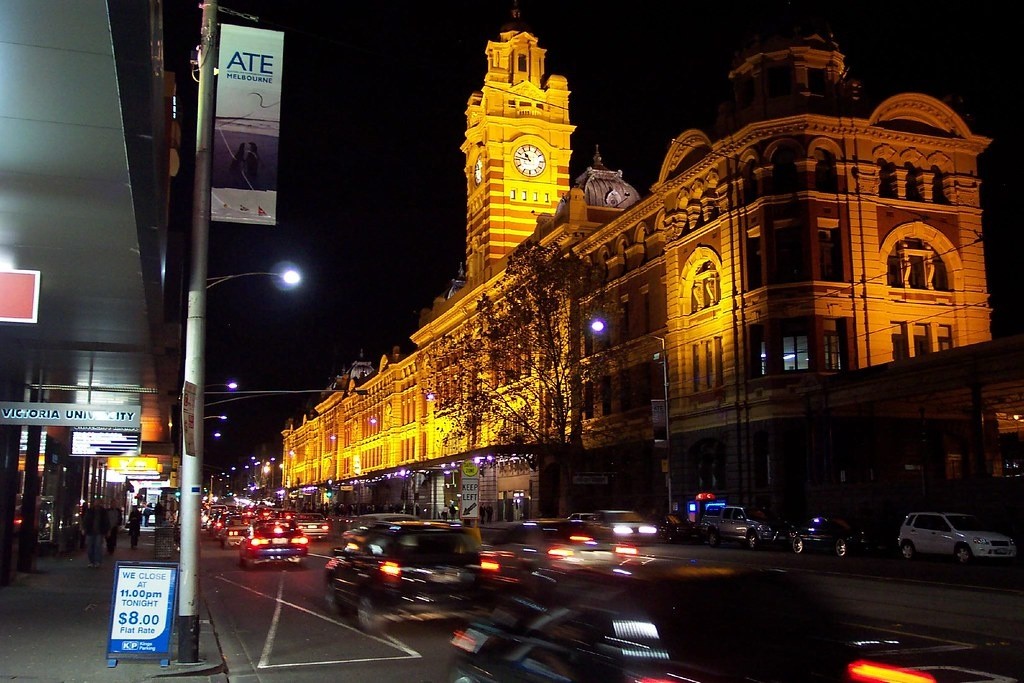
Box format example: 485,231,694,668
459,477,480,520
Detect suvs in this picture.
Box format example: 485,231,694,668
896,511,1018,568
698,503,780,550
322,519,483,631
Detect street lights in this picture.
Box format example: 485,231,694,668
591,319,673,514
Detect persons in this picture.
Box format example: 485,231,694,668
416,507,420,516
479,503,493,524
129,506,142,549
450,505,456,521
82,499,122,567
337,503,388,516
141,501,164,527
443,505,448,520
325,502,328,508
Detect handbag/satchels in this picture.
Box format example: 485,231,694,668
125,522,130,527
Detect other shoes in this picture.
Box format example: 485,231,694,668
88,562,94,568
95,562,100,568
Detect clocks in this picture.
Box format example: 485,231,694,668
513,144,548,178
472,152,483,188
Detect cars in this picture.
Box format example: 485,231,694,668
202,505,422,569
489,518,641,562
787,515,866,559
645,512,695,545
452,555,1024,683
567,513,612,533
598,509,658,540
